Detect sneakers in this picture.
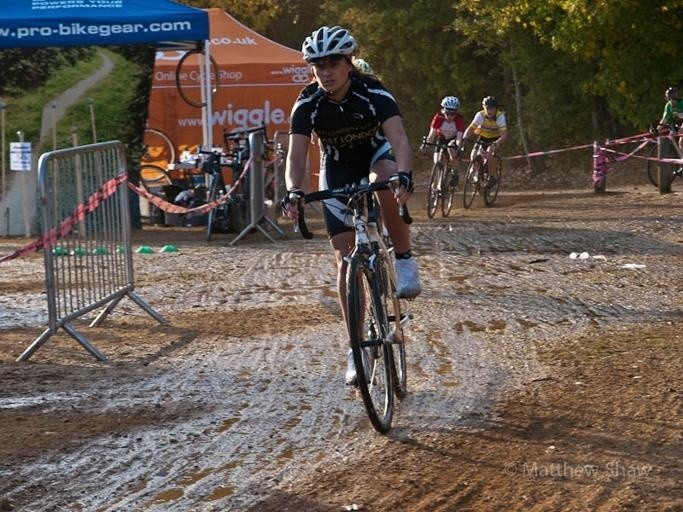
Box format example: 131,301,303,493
484,175,497,188
448,173,459,186
395,256,421,299
345,346,371,387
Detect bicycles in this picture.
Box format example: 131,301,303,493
196,125,251,241
645,120,682,190
421,135,464,219
284,173,415,436
461,136,502,210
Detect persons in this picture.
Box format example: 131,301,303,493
649,86,683,177
459,94,508,193
419,94,466,207
282,20,422,387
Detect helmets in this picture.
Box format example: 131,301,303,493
482,96,498,106
301,26,356,65
441,96,460,110
664,87,682,100
